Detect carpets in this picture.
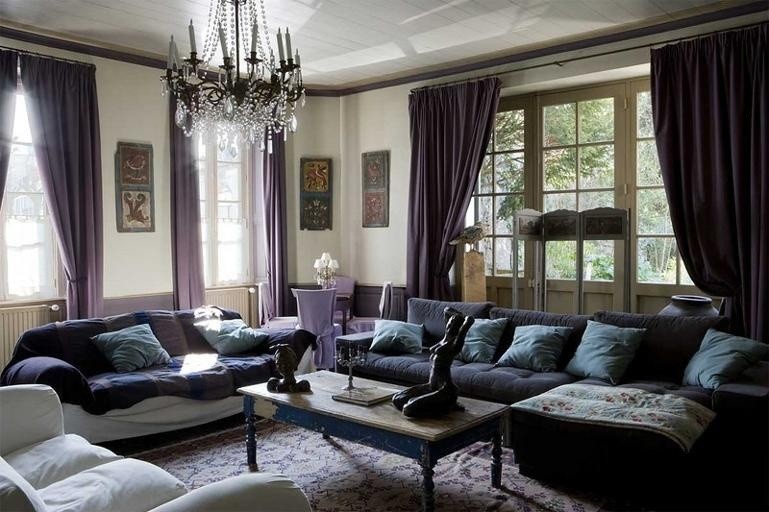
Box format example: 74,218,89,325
124,417,613,512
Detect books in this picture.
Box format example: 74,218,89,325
332,386,398,407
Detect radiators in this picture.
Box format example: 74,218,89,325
0,304,59,379
203,287,257,327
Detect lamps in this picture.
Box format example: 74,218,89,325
159,0,307,158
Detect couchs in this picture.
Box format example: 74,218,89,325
0,306,316,456
0,380,313,512
334,299,769,446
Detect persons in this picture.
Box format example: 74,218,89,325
392,305,476,417
266,343,310,393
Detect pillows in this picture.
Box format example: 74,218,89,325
190,318,267,356
369,320,426,354
566,319,649,386
682,329,769,387
497,325,576,374
455,318,508,362
91,322,173,374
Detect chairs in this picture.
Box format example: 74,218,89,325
346,281,394,335
257,282,299,331
289,289,344,371
329,276,354,326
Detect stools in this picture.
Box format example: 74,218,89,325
513,384,718,505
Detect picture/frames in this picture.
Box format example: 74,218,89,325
300,157,332,231
361,151,390,227
115,141,155,233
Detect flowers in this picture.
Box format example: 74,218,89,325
314,253,339,289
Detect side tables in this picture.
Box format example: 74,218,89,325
333,293,352,335
